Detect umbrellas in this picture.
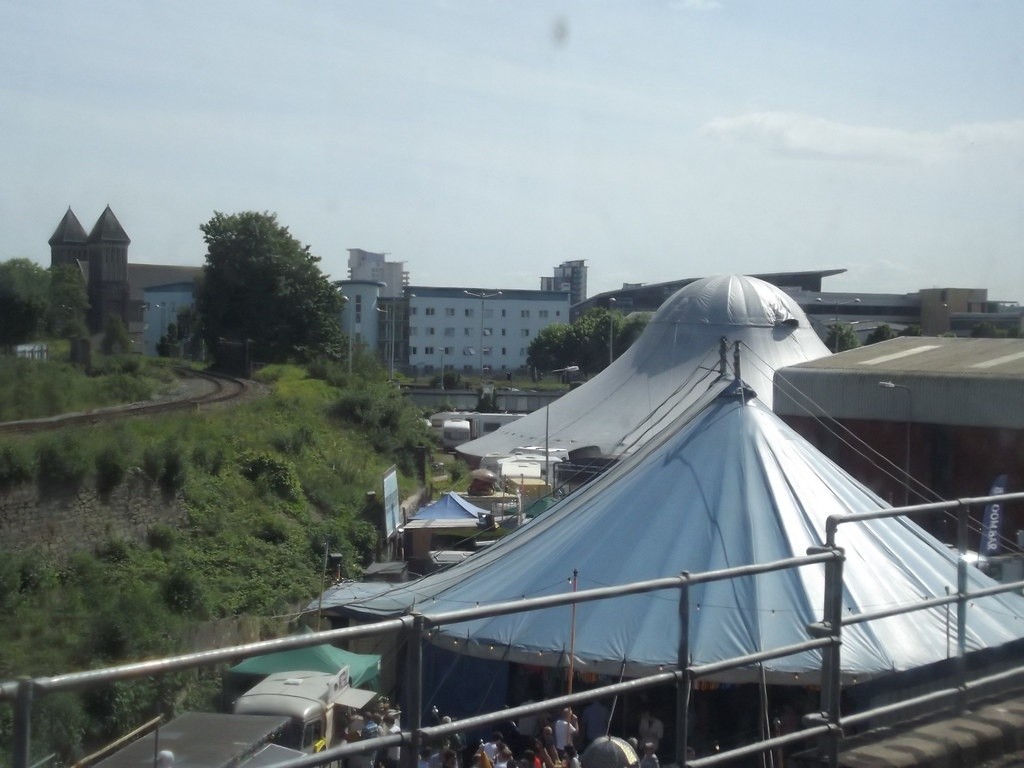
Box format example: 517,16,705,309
579,735,640,768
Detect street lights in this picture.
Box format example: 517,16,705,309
879,381,912,506
815,297,862,352
609,297,615,365
389,293,416,379
463,289,502,379
546,365,579,495
438,348,445,386
342,296,352,374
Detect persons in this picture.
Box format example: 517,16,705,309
579,688,610,741
418,716,462,768
506,369,516,383
626,709,664,768
468,703,579,768
346,711,404,768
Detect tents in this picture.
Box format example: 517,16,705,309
217,625,383,692
404,492,492,521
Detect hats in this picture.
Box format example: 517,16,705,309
419,746,434,755
442,716,451,723
